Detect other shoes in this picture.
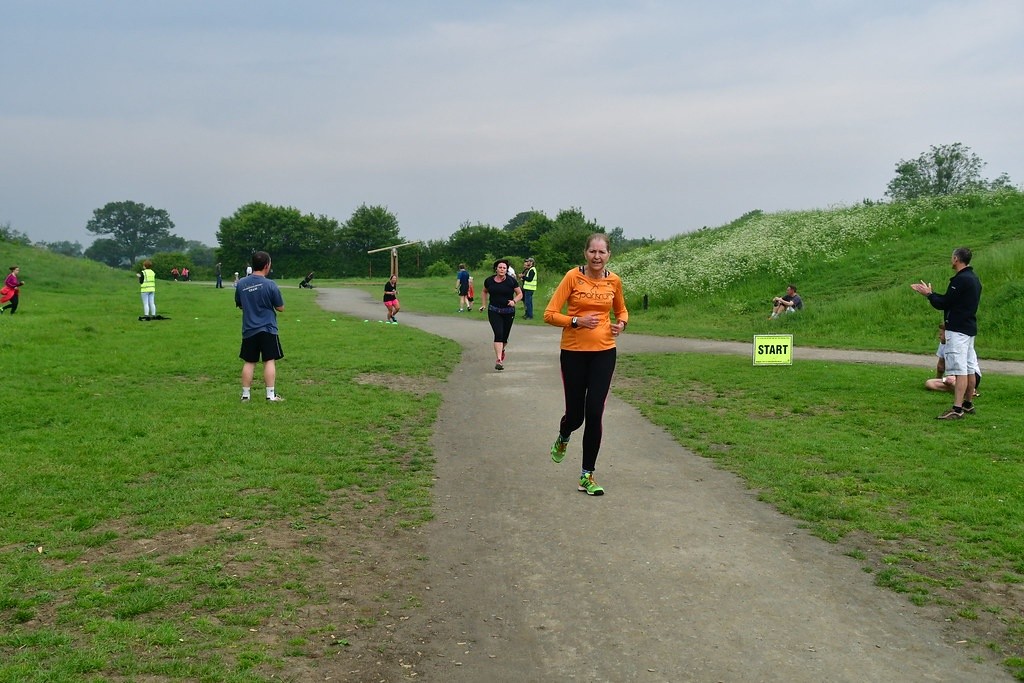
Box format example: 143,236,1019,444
387,320,393,323
391,316,397,322
241,397,249,402
457,309,464,313
267,393,283,401
0,307,4,314
467,307,471,311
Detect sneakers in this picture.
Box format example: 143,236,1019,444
495,360,504,370
551,435,571,463
963,407,975,415
502,350,505,360
935,408,964,421
577,473,604,496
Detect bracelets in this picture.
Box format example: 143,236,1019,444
512,300,516,303
571,317,579,329
622,321,627,331
455,287,457,289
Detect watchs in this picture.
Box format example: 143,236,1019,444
926,291,933,296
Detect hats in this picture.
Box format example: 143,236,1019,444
524,258,535,263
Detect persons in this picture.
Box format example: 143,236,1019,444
182,267,192,281
171,267,179,281
924,326,983,397
468,276,475,310
216,263,224,288
543,232,628,496
0,266,25,314
136,260,157,318
234,250,285,403
299,273,315,289
383,274,401,323
519,256,538,320
246,262,253,277
910,248,981,419
768,285,803,319
455,263,472,313
478,259,523,370
